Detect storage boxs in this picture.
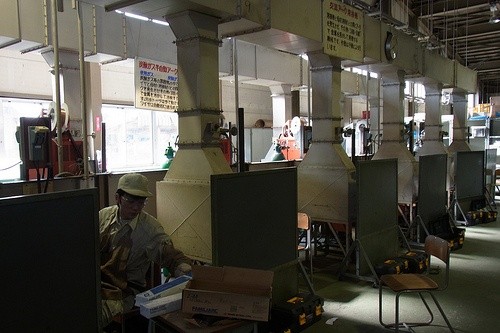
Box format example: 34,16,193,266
135,263,275,323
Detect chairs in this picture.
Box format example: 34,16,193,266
379,236,456,333
296,211,315,295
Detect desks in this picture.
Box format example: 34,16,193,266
152,310,257,333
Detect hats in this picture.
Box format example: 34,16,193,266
117,174,151,197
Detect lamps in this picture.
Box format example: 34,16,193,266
488,1,500,24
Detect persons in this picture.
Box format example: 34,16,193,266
255,119,265,128
98,174,185,328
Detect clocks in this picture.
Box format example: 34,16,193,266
384,31,398,61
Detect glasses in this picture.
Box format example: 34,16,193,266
120,196,146,209
